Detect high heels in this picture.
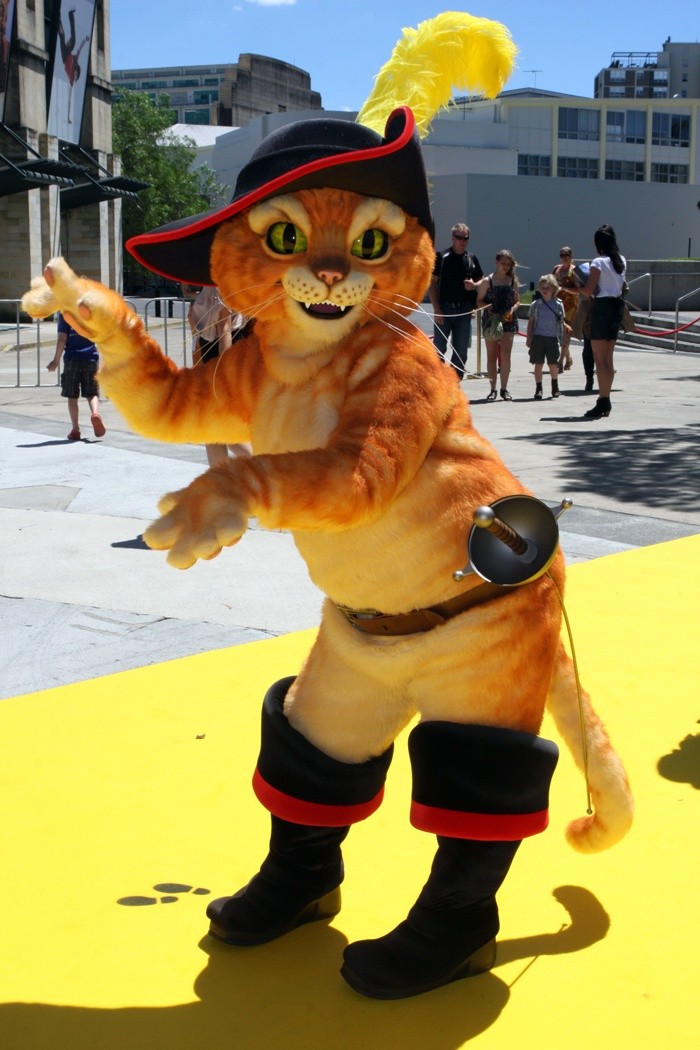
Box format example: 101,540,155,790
585,398,612,417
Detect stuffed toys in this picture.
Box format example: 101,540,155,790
21,12,634,1000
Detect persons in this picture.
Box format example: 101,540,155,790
525,272,565,400
183,285,256,468
59,9,90,125
476,249,519,403
576,224,627,417
429,223,483,380
552,246,617,393
46,310,107,440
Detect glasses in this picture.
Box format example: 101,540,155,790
452,234,469,240
560,254,570,258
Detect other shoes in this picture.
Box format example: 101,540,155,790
534,391,543,399
91,413,106,437
500,389,512,400
487,389,497,400
585,376,594,391
67,430,80,440
552,388,559,397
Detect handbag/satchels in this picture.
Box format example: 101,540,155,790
482,313,503,342
